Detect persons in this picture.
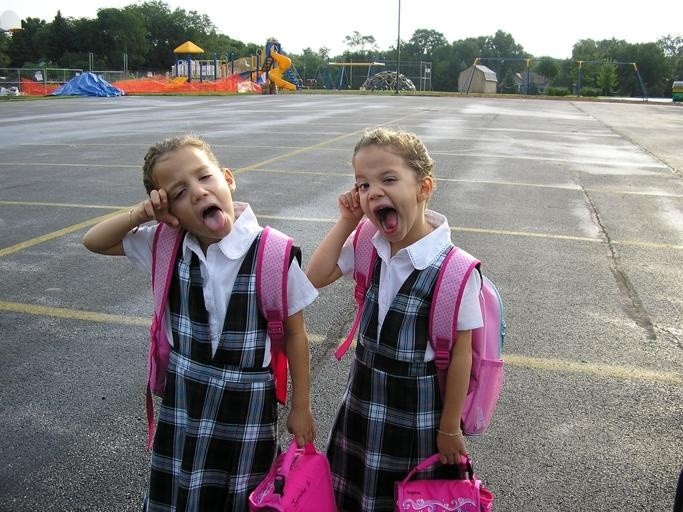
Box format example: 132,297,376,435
300,128,486,512
79,132,323,508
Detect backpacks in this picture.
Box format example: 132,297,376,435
335,219,504,435
145,220,294,406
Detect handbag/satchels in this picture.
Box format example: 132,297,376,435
394,453,494,512
248,437,337,512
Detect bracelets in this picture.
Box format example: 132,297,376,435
434,429,463,438
126,207,140,235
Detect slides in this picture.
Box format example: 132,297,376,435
268,48,295,90
170,76,187,86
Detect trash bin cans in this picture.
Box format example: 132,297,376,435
672,80,683,102
